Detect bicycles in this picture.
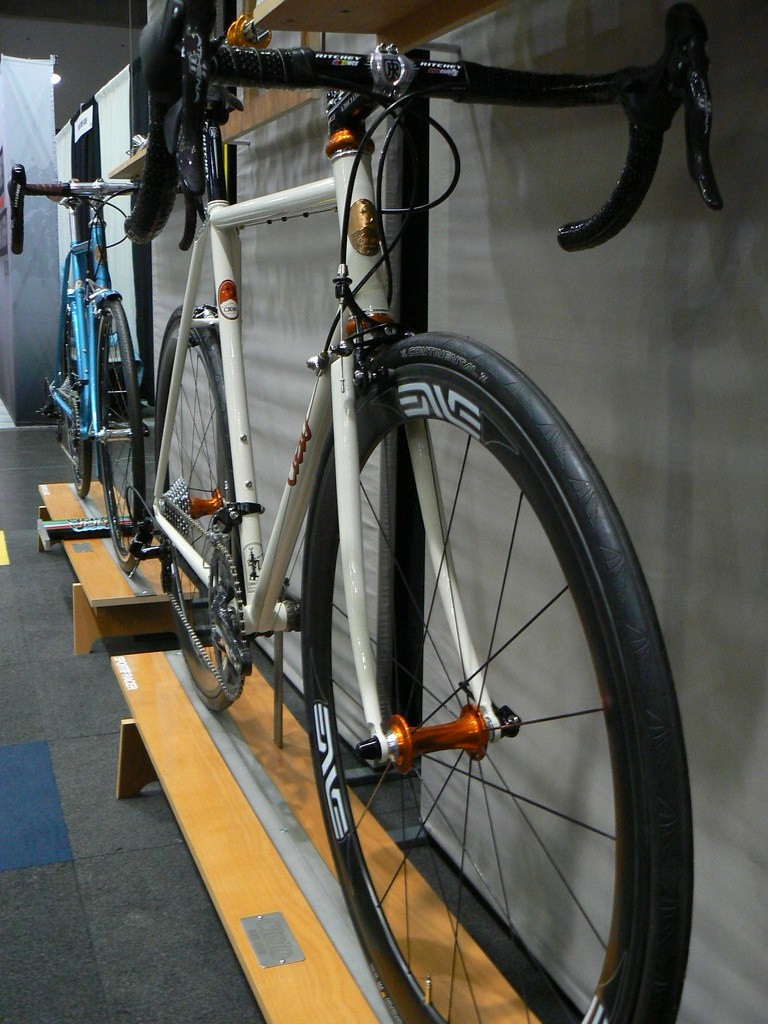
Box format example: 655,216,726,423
7,164,145,574
125,0,725,1024
